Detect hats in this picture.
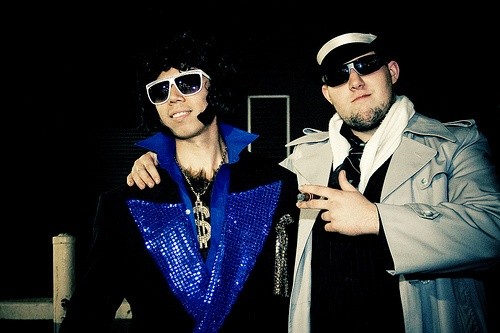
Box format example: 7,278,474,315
316,31,380,72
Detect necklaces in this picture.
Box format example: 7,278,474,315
346,155,362,175
173,148,227,249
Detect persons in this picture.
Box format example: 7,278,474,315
125,22,500,333
58,29,299,333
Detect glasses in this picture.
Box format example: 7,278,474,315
320,50,387,87
145,68,212,105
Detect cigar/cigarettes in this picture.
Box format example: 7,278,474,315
297,193,321,201
